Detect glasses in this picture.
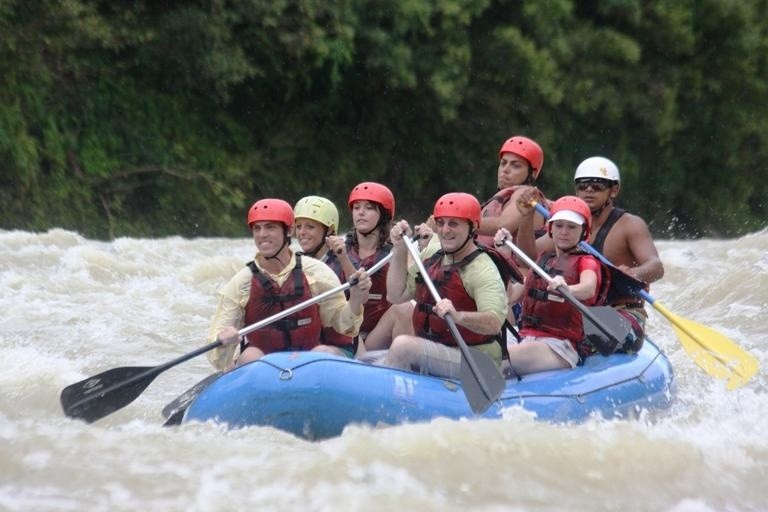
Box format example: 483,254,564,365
435,220,469,229
576,178,611,192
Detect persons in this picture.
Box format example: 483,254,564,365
425,133,549,325
293,194,359,303
331,181,416,349
205,197,372,368
494,195,602,377
380,191,510,379
515,156,664,356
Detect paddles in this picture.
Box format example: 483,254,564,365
162,368,226,428
531,201,761,392
60,278,359,425
502,237,632,357
402,231,506,414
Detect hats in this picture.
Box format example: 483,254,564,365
548,211,586,227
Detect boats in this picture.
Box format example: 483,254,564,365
179,332,680,446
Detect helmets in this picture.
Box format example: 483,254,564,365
433,192,481,230
574,155,621,188
247,198,295,228
499,136,544,178
293,194,340,235
347,182,394,224
548,195,592,243
